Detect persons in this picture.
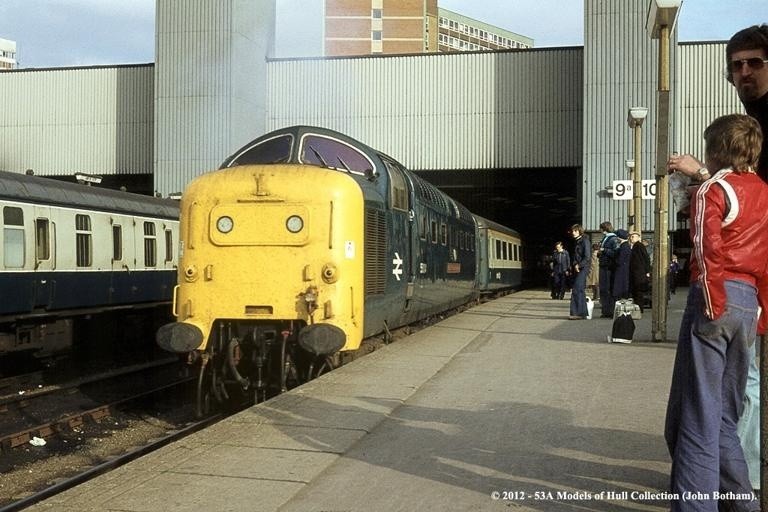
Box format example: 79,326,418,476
661,114,767,512
662,23,768,497
549,240,570,299
628,231,651,313
670,254,679,294
610,227,630,302
597,221,617,318
586,243,600,300
568,224,592,320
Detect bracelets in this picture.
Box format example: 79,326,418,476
693,166,710,181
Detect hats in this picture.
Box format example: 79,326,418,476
628,231,640,236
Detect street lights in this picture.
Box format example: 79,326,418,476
625,159,635,233
643,0,682,347
627,108,649,244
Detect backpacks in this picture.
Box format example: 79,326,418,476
611,313,635,344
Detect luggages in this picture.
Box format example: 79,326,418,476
612,298,641,320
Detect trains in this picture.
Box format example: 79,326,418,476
157,126,529,419
1,168,180,368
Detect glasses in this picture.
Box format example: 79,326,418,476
727,57,768,72
